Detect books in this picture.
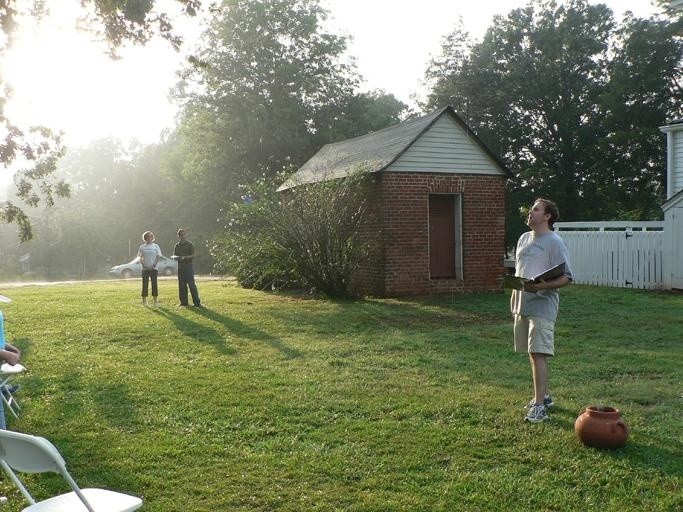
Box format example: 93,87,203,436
501,259,566,294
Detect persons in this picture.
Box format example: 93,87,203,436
173,228,200,307
0,310,21,430
508,197,573,423
137,230,161,308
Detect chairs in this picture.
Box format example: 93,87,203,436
0,374,21,420
0,429,143,512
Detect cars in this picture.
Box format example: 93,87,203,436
109,254,178,278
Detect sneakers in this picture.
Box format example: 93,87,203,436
523,393,554,424
